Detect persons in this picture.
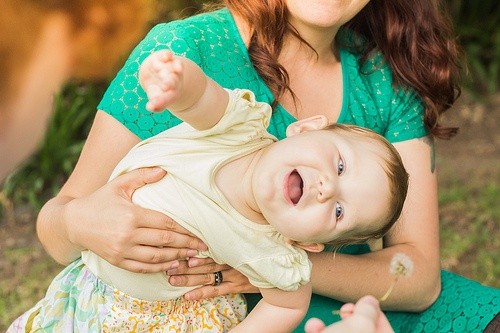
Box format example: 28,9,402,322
36,0,500,333
5,50,409,333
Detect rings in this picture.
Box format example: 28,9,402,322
213,271,223,286
